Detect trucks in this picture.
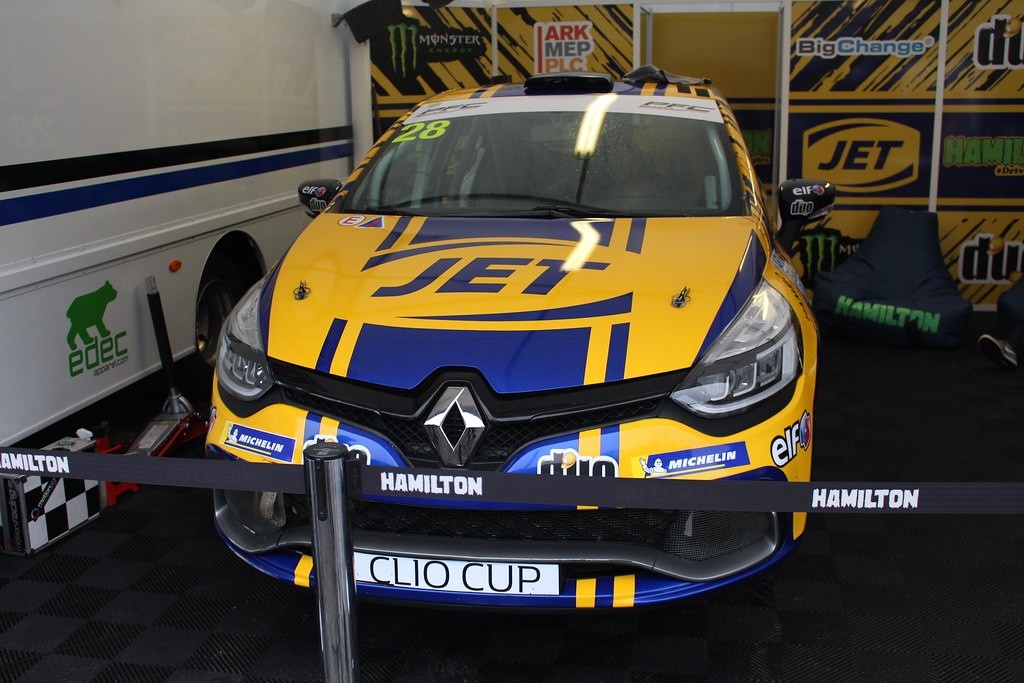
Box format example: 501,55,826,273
0,0,378,557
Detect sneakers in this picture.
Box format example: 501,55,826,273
979,334,1018,367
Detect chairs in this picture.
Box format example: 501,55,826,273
809,204,976,345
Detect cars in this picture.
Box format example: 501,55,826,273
204,72,839,610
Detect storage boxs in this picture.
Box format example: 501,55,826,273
0,437,103,556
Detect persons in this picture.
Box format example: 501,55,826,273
977,321,1024,371
